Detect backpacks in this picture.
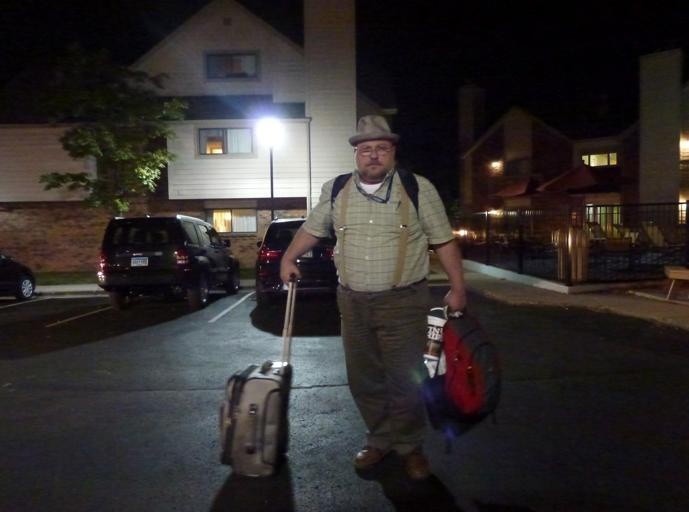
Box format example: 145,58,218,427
422,308,509,436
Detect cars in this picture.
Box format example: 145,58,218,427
0,254,35,299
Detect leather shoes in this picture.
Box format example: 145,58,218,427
406,448,429,481
357,442,392,464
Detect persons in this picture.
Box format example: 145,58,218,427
279,115,466,483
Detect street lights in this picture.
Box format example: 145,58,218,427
253,114,285,221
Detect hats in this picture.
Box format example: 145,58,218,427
349,115,400,144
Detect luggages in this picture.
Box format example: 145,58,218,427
219,277,298,480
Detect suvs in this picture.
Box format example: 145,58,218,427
96,214,239,308
255,216,338,309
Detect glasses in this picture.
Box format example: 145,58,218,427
355,144,395,154
